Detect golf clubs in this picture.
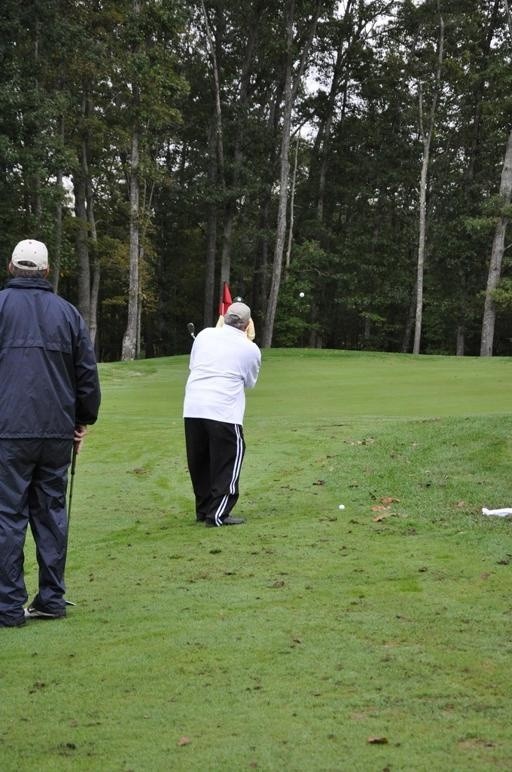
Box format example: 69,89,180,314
187,322,196,339
63,425,80,605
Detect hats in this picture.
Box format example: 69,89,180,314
225,296,250,327
11,239,49,271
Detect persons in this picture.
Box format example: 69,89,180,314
1,236,102,628
182,301,263,525
215,295,257,342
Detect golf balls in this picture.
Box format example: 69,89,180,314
339,505,347,509
300,292,306,298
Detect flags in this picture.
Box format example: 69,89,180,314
217,279,233,317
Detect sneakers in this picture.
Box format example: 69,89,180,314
22,607,66,620
197,513,244,527
